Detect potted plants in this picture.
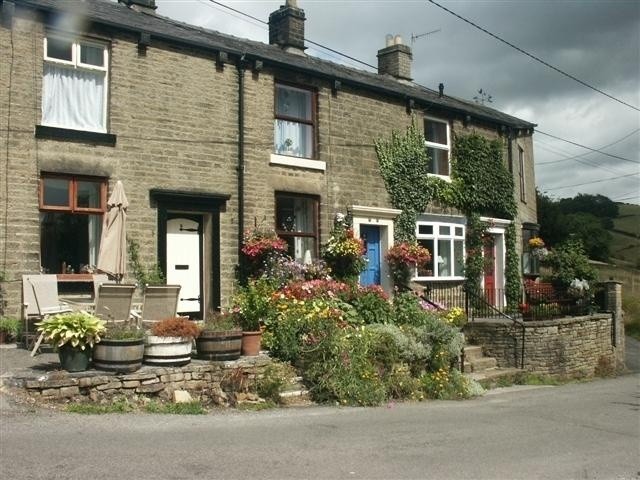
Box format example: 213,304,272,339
144,316,201,367
33,310,108,372
92,323,146,373
227,273,281,357
195,308,243,361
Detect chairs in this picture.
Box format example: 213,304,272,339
130,284,182,328
21,273,74,357
79,283,138,324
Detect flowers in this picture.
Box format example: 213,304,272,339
390,241,431,267
264,255,390,329
321,213,367,257
242,222,289,257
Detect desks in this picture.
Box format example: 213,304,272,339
59,297,146,306
